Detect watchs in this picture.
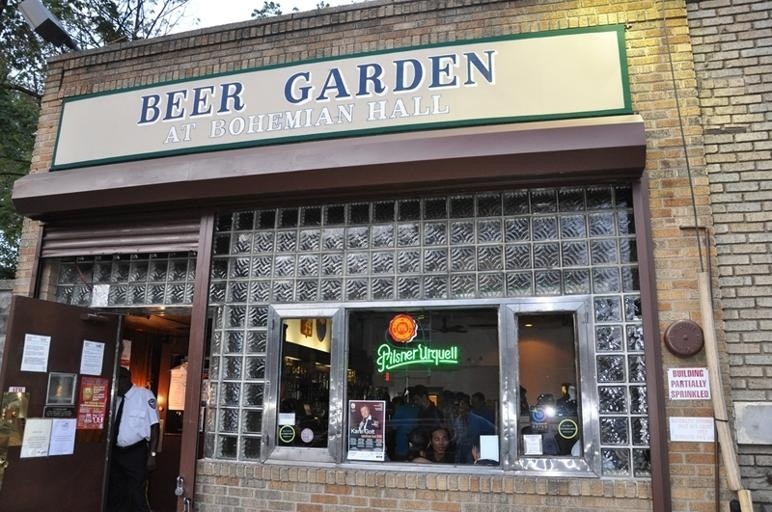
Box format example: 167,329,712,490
150,451,159,457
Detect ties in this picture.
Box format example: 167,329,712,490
113,397,125,444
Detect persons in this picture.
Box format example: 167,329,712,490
357,405,381,449
384,385,500,466
107,367,160,511
519,383,582,457
